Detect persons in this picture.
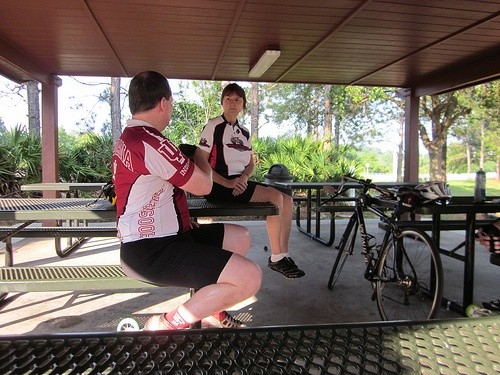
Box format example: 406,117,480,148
197,81,307,280
111,71,263,331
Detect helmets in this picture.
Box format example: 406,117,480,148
415,181,452,205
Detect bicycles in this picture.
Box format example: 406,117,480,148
327,174,444,322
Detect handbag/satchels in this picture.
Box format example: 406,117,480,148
101,180,116,205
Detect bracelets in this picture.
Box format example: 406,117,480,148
243,173,250,177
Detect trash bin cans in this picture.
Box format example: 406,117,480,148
264,164,293,197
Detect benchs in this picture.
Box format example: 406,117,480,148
314,206,385,212
0,228,118,238
0,265,156,292
293,197,355,201
379,220,496,231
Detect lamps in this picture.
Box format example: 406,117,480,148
248,49,281,78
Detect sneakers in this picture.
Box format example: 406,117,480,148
268,256,305,279
144,313,192,330
200,310,246,328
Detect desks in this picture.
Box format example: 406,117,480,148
0,182,500,375
